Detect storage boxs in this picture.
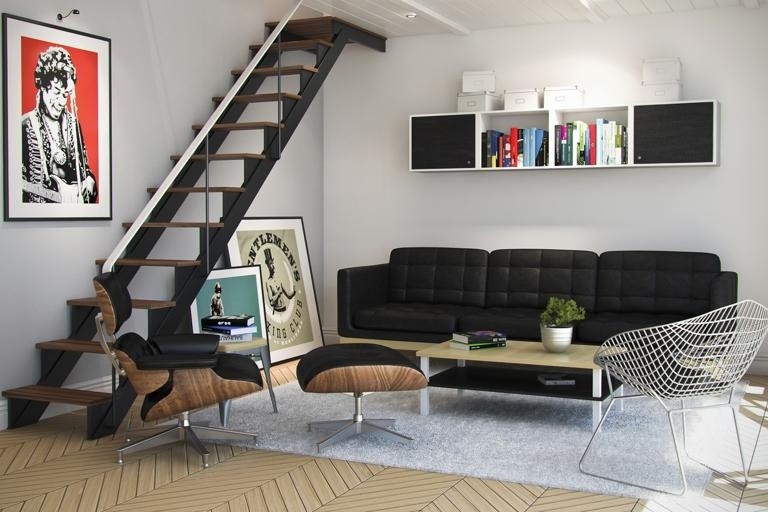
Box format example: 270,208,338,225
642,57,683,81
456,69,585,113
641,83,684,102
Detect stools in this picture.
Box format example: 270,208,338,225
296,343,428,454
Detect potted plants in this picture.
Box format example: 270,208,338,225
539,296,585,352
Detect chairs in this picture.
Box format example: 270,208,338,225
93,272,263,469
578,299,768,497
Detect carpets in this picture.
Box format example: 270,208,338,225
155,356,751,508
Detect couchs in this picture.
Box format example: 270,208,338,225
337,247,738,351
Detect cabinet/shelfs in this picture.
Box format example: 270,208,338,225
408,99,720,172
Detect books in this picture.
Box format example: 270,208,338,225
448,339,506,350
200,315,257,342
481,118,626,168
452,330,506,344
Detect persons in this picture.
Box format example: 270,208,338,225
18,44,99,205
210,281,224,317
263,248,296,313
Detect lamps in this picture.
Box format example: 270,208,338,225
57,9,80,21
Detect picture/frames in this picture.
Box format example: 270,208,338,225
220,217,326,370
190,264,272,371
2,14,111,221
